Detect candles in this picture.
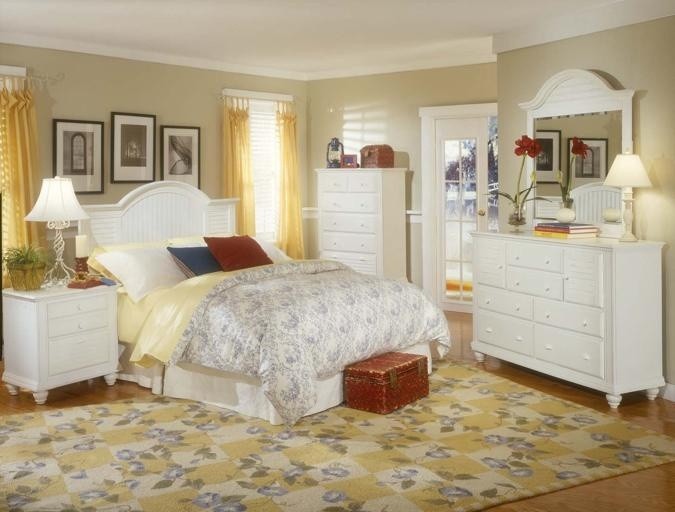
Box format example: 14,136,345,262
74,234,90,258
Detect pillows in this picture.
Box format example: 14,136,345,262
96,233,290,303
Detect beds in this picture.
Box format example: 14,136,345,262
77,182,454,424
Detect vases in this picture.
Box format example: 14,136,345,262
555,199,575,223
508,205,527,232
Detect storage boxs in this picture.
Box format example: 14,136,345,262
360,145,394,168
344,351,429,415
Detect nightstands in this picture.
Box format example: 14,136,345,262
2,282,119,404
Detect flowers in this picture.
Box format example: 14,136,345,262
482,134,551,218
556,138,589,209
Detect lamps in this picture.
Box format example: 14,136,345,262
603,154,653,243
23,175,90,287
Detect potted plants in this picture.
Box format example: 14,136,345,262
1,248,50,289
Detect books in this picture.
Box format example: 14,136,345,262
535,222,599,239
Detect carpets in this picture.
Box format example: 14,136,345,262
0,359,675,512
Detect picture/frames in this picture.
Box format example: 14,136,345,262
567,137,609,192
160,125,201,189
536,129,561,184
52,119,104,195
110,111,156,183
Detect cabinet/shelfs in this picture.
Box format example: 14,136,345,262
469,232,667,408
314,166,408,280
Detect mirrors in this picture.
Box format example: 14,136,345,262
518,69,636,237
